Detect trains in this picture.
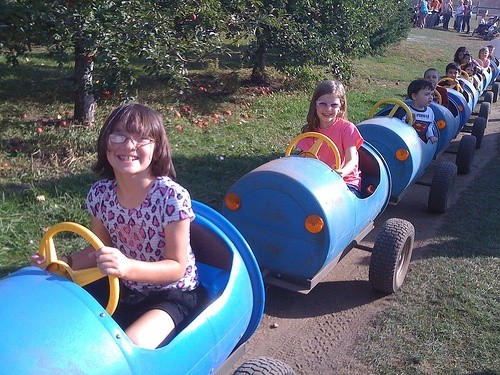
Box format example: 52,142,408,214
0,68,500,375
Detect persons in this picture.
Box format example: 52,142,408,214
398,0,500,144
32,103,201,349
295,80,366,198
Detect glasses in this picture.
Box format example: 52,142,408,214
109,134,156,146
316,101,341,108
